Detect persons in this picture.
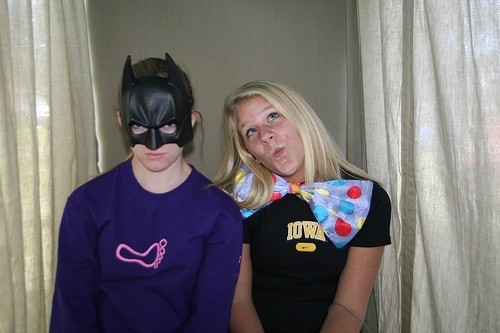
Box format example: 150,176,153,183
203,80,391,333
49,53,246,333
